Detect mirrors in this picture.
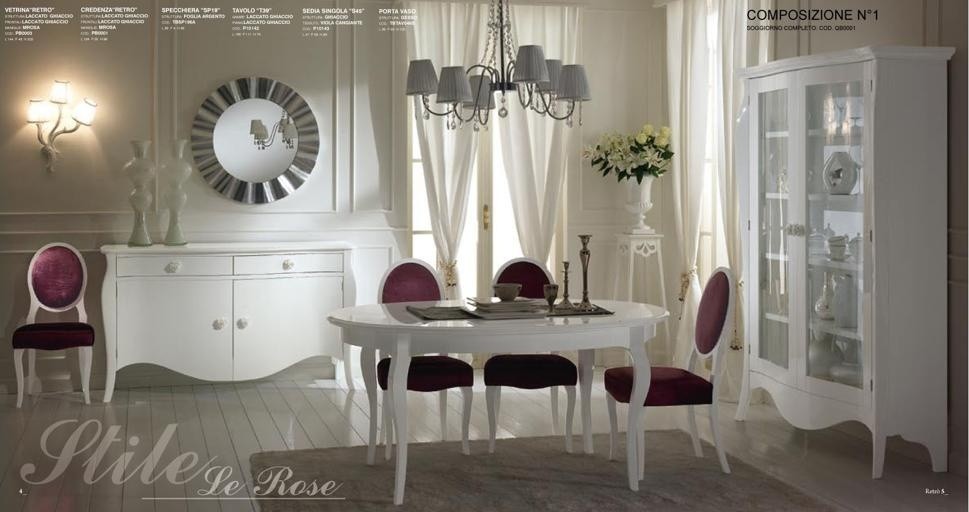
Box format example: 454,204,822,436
191,76,319,203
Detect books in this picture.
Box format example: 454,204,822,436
406,296,547,321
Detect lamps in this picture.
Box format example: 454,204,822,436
403,0,591,134
24,79,98,174
251,108,299,150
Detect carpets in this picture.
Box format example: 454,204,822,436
248,426,833,511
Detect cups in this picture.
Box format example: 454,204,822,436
493,282,522,301
809,230,846,253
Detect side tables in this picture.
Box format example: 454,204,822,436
326,296,669,505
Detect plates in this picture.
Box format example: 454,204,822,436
459,296,548,319
826,254,851,260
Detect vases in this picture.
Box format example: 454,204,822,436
123,133,194,247
618,174,657,237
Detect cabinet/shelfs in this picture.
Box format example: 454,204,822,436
616,235,673,367
98,242,358,405
734,46,956,481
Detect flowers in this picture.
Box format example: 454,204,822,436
580,122,675,187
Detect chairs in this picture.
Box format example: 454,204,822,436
376,257,475,460
483,255,577,456
11,240,95,411
603,265,736,493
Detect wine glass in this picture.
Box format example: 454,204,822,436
543,284,557,313
